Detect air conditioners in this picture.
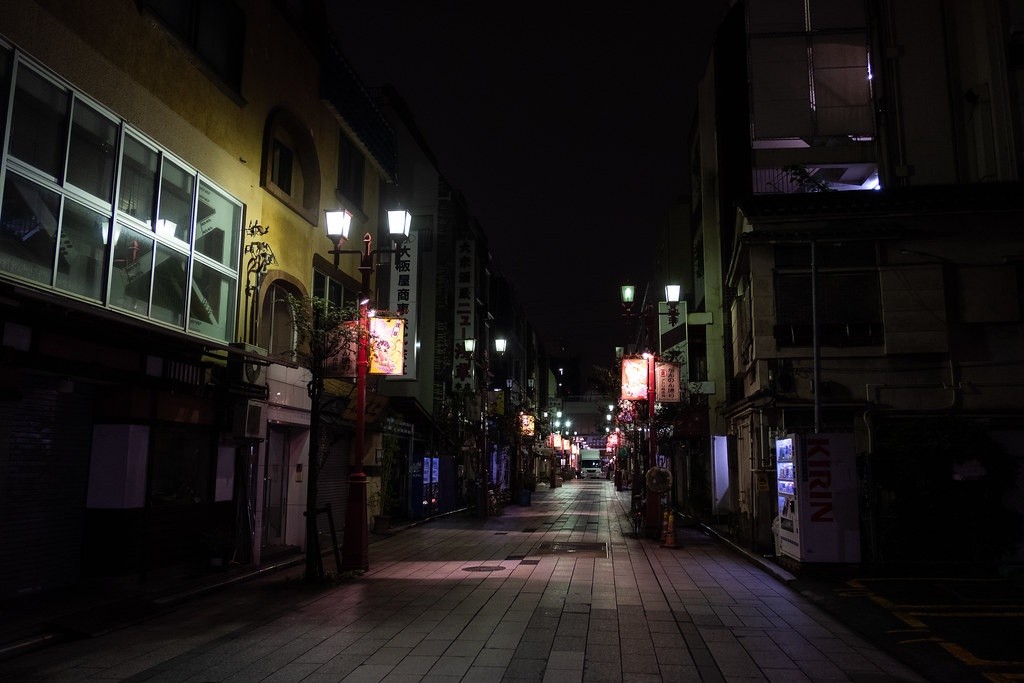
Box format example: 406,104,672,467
232,399,268,439
227,342,268,388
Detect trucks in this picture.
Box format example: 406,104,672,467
576,448,602,479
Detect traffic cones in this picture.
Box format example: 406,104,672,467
663,514,679,547
659,512,669,544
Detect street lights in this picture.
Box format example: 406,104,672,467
464,335,507,515
325,205,414,574
619,282,682,535
543,410,563,487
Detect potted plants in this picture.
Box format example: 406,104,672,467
374,432,400,535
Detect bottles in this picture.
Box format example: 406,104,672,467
778,445,793,493
